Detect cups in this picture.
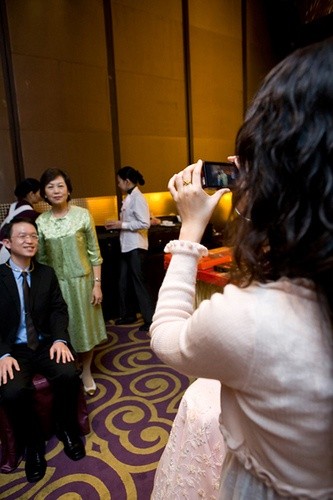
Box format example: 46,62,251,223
104,219,113,230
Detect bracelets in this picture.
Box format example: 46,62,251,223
93,277,101,282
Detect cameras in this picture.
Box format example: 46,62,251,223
200,161,240,189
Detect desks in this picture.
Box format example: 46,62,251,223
94,216,214,317
161,246,246,302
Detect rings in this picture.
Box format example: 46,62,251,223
183,180,192,186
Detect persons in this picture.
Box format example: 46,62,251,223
215,168,231,185
150,38,333,500
0,177,42,266
0,218,86,483
104,166,154,332
33,167,108,396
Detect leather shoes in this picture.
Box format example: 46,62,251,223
25,447,48,483
56,429,85,462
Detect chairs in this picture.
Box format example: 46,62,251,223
1,374,91,465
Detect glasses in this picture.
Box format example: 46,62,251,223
10,232,39,242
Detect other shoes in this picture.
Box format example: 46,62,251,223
83,378,97,397
140,321,150,332
115,315,138,325
